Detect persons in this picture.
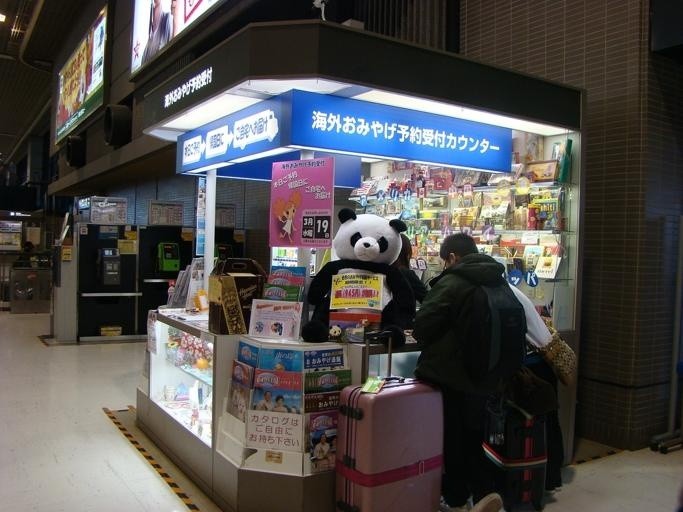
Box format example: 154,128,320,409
412,234,526,511
255,390,274,410
141,1,179,69
392,233,427,329
271,395,291,413
501,276,564,497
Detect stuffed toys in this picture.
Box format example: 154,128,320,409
301,208,415,349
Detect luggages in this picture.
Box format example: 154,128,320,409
335,330,446,511
479,395,549,512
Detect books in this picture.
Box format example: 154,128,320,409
226,338,261,422
253,367,303,391
250,265,306,340
303,349,352,473
168,256,218,310
365,157,562,280
251,389,303,415
258,347,304,372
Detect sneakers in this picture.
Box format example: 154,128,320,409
468,490,502,512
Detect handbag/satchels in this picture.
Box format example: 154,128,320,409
536,325,579,387
514,363,560,418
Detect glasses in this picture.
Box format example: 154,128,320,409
443,258,447,270
